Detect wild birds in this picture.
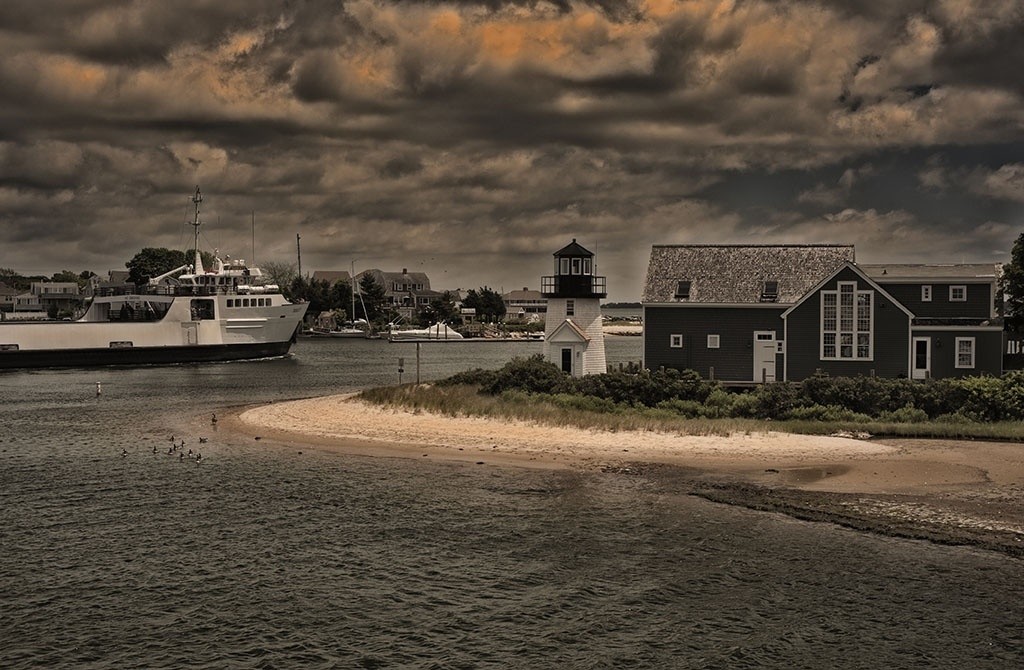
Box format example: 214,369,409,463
121,411,217,465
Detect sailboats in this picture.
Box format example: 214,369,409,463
329,261,372,338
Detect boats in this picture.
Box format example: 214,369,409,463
377,313,464,340
0,187,312,370
